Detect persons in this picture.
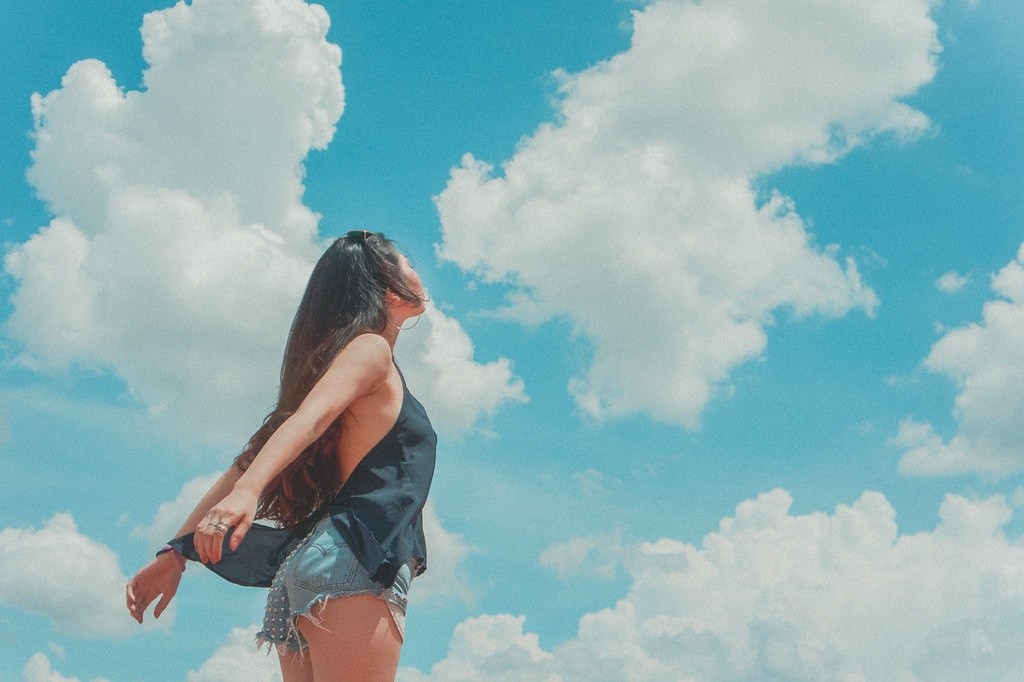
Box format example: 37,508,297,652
126,230,438,682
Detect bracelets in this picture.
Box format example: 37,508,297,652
156,546,185,572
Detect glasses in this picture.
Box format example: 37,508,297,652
346,230,374,245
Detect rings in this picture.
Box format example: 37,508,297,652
208,523,216,527
219,521,229,528
215,526,225,534
